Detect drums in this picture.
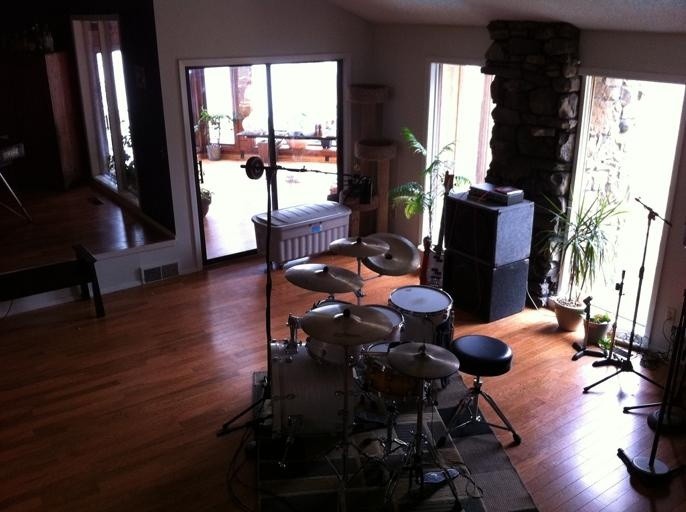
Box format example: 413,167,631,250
269,342,353,438
309,300,363,363
388,284,453,347
366,341,415,394
360,304,404,347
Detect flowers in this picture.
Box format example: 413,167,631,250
596,329,614,349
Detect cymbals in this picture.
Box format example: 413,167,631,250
359,233,418,275
302,302,391,343
328,236,389,259
388,342,459,378
284,262,364,293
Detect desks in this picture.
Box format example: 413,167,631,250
237,130,337,162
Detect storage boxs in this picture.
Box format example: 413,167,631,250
251,202,353,263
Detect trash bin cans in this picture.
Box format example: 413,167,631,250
206,145,222,161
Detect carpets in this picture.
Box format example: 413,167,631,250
253,369,539,512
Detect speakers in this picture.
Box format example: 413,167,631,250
444,192,534,268
444,249,530,323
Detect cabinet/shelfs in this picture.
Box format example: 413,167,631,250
0,52,85,189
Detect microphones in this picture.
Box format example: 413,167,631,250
359,180,372,204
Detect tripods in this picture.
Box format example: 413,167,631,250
222,168,272,433
308,344,383,512
383,380,467,512
623,282,686,413
364,400,408,459
584,216,667,393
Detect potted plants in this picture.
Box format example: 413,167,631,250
194,111,234,161
539,193,627,331
124,162,136,185
340,171,376,205
200,189,212,217
584,313,610,345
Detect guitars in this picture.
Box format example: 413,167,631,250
418,171,454,285
